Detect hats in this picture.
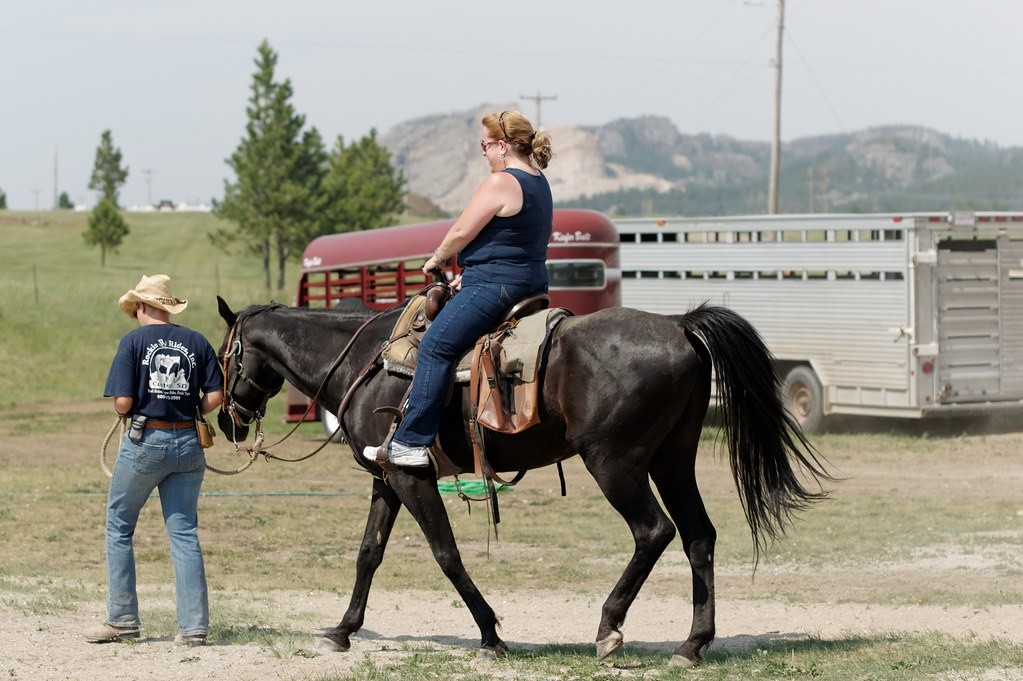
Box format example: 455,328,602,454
118,274,188,318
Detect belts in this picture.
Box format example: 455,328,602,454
145,421,194,430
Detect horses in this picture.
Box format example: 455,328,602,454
216,295,853,670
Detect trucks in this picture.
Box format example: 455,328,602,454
612,210,1023,434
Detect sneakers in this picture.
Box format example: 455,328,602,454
83,622,140,642
174,633,206,647
363,441,429,467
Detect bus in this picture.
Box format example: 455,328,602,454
286,209,621,444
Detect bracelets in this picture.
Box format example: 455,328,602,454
434,247,444,262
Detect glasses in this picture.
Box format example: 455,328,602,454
480,140,508,156
132,302,144,319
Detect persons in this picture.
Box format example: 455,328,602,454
83,274,224,647
362,109,553,468
